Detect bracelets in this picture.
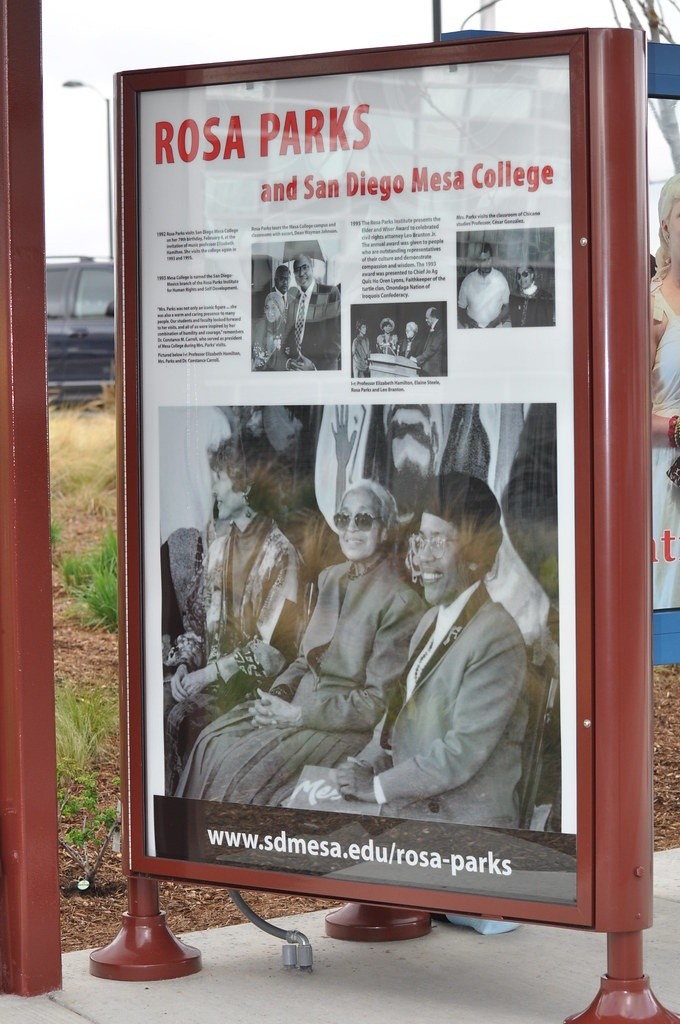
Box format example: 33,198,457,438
668,416,680,449
346,756,369,771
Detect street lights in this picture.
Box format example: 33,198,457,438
61,80,114,255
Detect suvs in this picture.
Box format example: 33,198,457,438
45,256,115,386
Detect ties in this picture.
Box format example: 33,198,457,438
297,294,306,348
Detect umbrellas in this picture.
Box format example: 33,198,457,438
252,240,325,296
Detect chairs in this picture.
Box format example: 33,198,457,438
283,503,552,826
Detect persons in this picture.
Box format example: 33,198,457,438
510,264,554,328
409,307,442,376
352,318,371,378
253,255,342,370
334,472,531,830
165,430,305,798
178,476,427,801
401,322,424,361
458,241,510,328
652,174,680,666
377,318,399,356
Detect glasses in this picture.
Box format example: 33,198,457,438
411,533,460,561
516,270,530,279
333,513,382,532
